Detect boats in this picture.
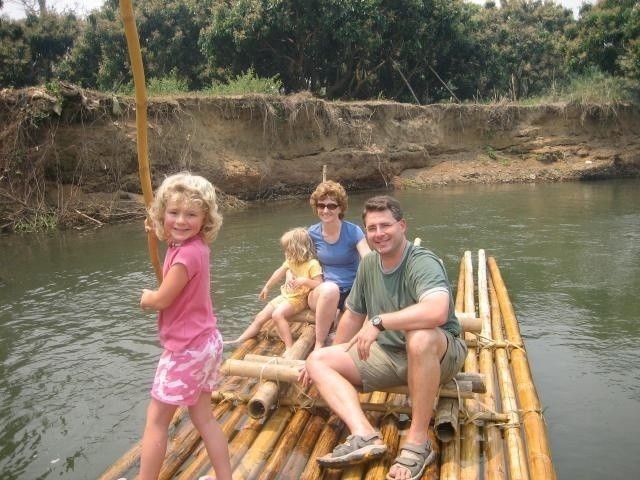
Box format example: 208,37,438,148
88,237,558,480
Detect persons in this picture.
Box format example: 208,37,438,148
115,170,233,480
222,225,324,357
284,179,374,353
296,195,470,480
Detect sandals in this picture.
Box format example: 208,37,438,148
314,431,388,469
384,436,437,480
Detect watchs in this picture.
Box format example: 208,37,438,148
370,316,385,332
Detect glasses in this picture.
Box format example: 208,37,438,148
315,202,341,210
